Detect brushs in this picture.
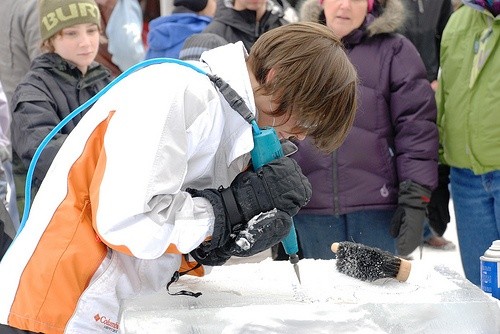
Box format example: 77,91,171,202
330,238,412,285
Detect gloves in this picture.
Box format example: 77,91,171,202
390,181,431,257
192,208,292,265
427,182,450,236
184,157,312,251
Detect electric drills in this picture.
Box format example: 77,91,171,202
248,126,304,285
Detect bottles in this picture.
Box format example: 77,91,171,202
479,239,500,299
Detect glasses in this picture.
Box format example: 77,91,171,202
296,113,319,129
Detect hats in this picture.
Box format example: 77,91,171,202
178,32,227,62
39,0,102,43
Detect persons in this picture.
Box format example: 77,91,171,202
288,0,437,262
204,0,296,55
330,241,465,293
430,0,500,291
145,0,218,67
94,0,146,79
1,0,49,116
11,0,125,225
393,0,459,253
181,32,231,65
1,21,360,334
